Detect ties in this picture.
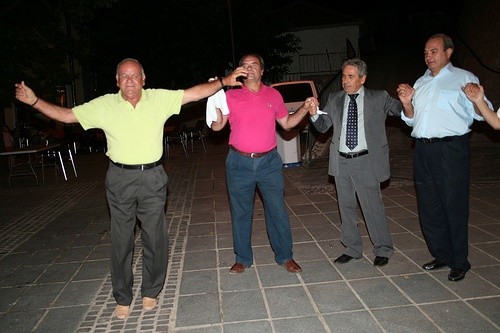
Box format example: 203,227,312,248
345,93,360,151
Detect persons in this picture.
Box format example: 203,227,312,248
308,59,412,268
16,58,250,320
208,55,319,275
397,34,494,281
466,85,500,130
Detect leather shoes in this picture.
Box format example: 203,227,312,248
422,259,447,271
373,256,389,267
228,263,247,275
282,259,302,273
334,254,356,265
447,269,468,282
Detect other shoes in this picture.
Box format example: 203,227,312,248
142,297,157,311
114,304,129,320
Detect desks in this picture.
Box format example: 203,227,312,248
0,143,61,186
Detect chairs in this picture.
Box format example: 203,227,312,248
18,127,105,181
164,120,208,159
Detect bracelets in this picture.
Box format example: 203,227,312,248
219,77,224,87
31,97,39,106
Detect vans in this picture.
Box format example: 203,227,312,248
270,81,320,115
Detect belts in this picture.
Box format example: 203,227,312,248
414,133,470,144
337,149,368,160
108,157,162,170
228,144,276,159
